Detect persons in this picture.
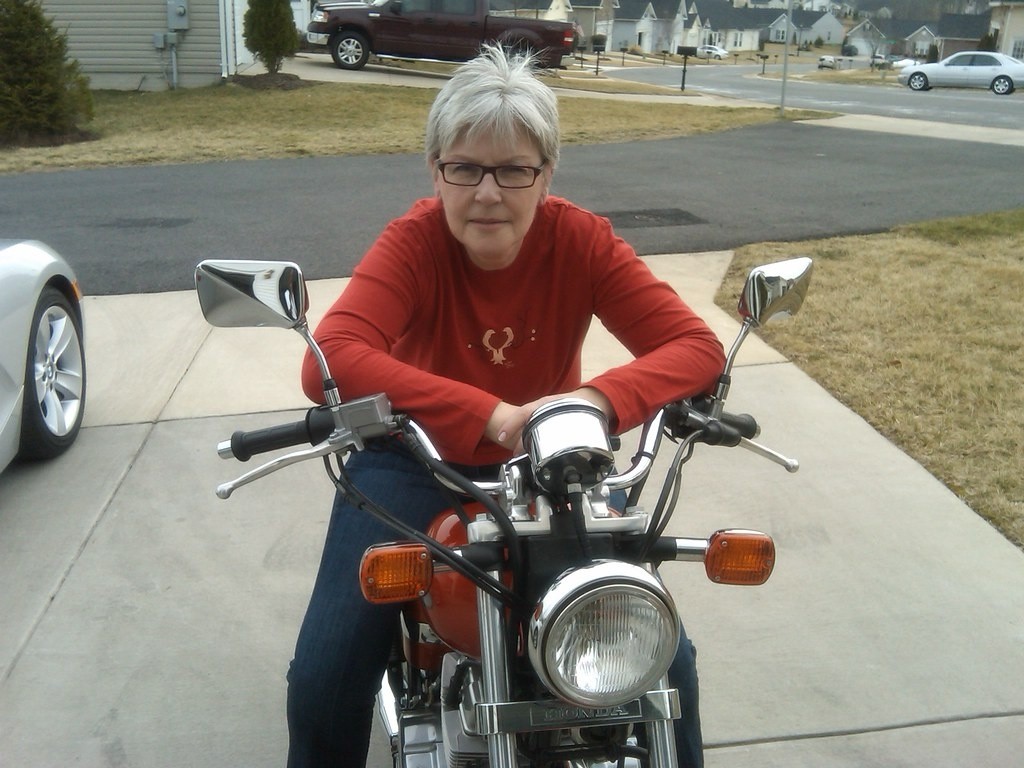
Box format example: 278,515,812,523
285,47,726,764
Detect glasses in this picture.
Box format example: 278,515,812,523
435,154,546,191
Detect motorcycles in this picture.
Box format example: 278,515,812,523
186,255,815,768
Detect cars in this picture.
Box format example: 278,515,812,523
817,55,835,70
697,45,728,62
897,51,1023,96
0,240,88,464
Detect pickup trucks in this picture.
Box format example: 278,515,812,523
305,0,576,67
